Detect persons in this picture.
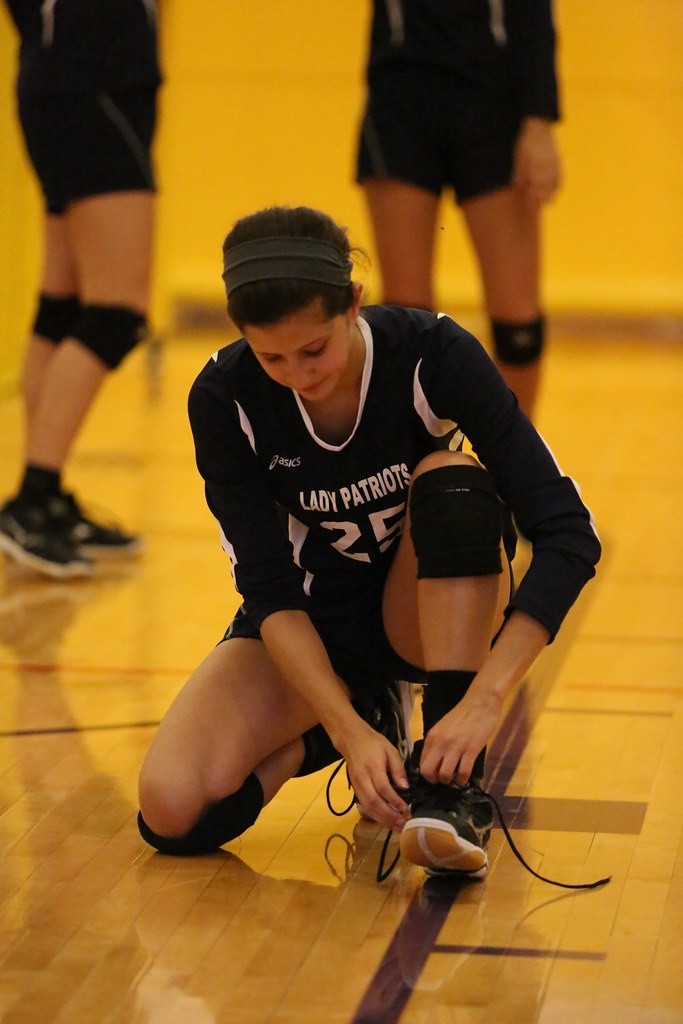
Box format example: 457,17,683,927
138,208,602,876
0,1,163,578
357,0,562,416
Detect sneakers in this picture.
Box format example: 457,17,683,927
0,492,143,581
375,739,584,889
325,678,412,820
